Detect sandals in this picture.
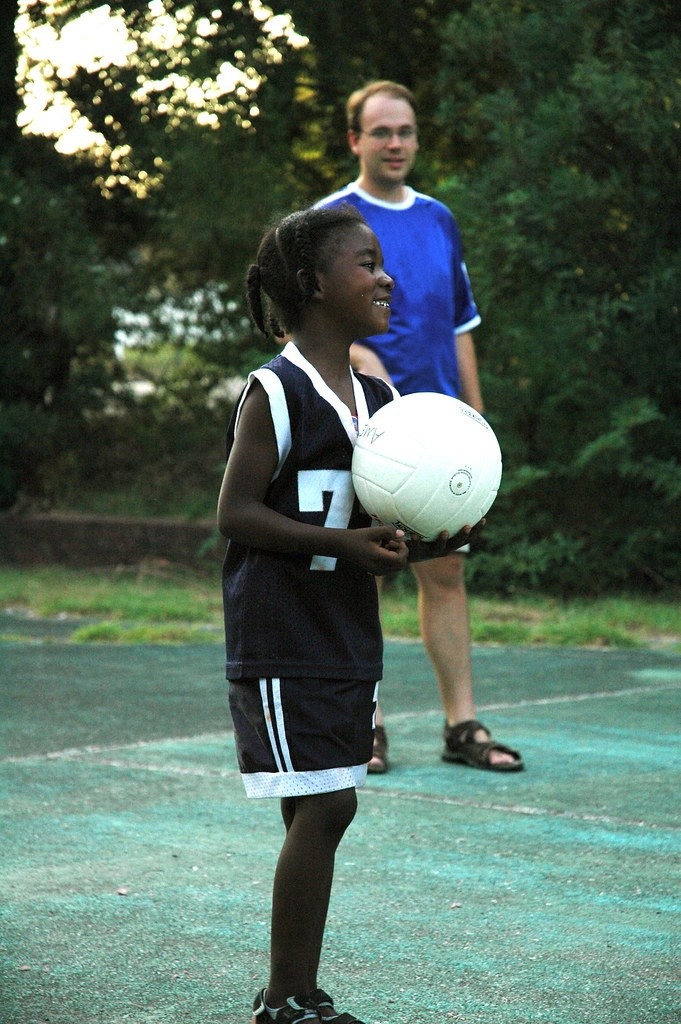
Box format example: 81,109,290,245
368,727,389,772
442,717,523,771
250,988,366,1024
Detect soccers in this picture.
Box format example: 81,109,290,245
352,390,504,544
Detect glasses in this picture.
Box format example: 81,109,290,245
359,127,422,144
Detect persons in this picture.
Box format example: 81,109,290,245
216,202,487,1024
306,80,525,773
